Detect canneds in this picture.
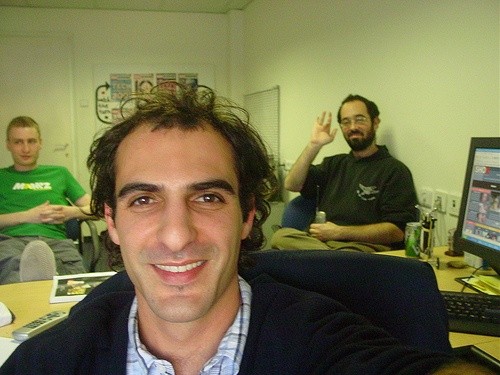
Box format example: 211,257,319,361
405,222,421,257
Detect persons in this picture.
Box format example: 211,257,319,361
269,94,417,254
0,82,494,375
0,115,100,284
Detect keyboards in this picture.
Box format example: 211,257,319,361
436,290,500,336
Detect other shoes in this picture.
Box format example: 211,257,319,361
19,240,56,283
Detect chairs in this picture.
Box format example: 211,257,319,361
70,247,452,356
64,199,100,274
280,195,315,231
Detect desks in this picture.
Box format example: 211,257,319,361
0,280,81,343
370,245,500,371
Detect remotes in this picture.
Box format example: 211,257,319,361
11,310,69,342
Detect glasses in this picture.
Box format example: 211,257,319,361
340,117,371,129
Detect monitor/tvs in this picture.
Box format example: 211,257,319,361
454,136,500,296
